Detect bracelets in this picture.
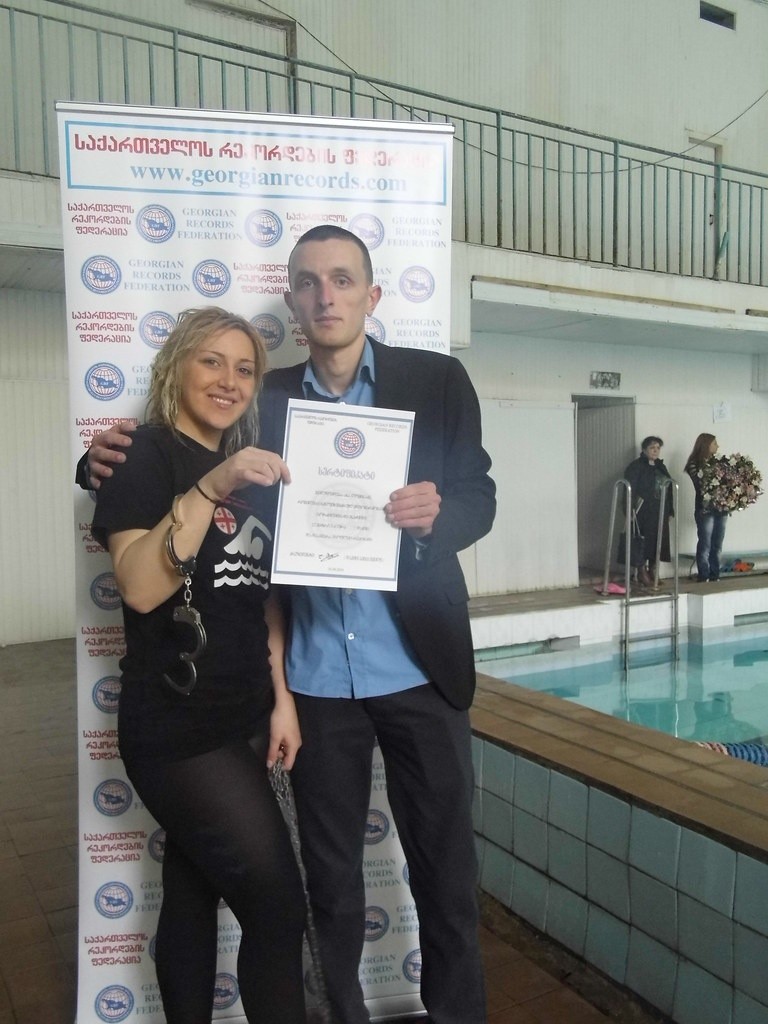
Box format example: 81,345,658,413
194,480,224,508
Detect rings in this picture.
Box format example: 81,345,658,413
281,746,284,749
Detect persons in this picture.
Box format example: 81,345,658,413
75,225,495,1023
92,306,307,1023
684,433,727,582
622,436,675,587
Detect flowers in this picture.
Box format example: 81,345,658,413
697,452,766,516
